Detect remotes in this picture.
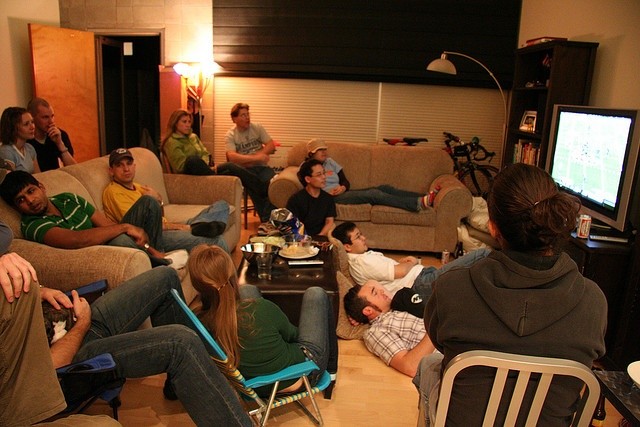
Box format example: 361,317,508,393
288,259,325,265
590,235,630,243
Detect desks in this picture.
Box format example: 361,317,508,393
593,370,640,427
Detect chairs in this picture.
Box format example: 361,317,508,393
161,137,173,175
418,349,601,427
170,289,331,427
38,278,124,427
225,150,269,229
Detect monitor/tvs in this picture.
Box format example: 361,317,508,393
544,104,640,232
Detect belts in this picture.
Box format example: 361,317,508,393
299,344,314,361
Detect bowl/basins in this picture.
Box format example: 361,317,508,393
240,243,281,265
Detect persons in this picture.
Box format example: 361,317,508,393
0,107,42,174
102,147,234,257
342,281,443,378
331,224,496,307
413,164,611,427
39,265,254,425
1,221,122,427
224,103,280,224
26,97,77,171
164,109,272,221
287,160,337,245
301,137,442,216
188,244,337,403
0,171,173,268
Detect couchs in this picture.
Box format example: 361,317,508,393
269,141,473,259
0,147,243,334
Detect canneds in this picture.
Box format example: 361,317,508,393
577,214,592,239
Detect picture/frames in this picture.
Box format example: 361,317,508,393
519,110,537,133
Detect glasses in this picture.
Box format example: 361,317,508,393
351,231,364,242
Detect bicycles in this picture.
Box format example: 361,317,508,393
384,131,465,184
403,137,499,196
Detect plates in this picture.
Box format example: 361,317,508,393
278,245,320,260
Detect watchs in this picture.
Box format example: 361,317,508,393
142,243,151,253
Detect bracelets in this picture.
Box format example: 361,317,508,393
60,147,68,154
160,200,165,207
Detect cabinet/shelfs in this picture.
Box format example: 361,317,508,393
500,40,599,176
560,229,635,356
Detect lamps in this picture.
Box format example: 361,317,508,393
426,52,506,166
174,62,214,113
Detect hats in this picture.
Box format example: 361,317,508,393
307,138,328,154
390,287,425,318
110,149,133,165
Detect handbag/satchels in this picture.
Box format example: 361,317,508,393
468,196,491,233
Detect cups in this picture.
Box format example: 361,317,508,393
258,253,272,280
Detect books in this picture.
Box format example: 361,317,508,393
512,139,542,169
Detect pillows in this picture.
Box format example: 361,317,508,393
335,271,372,340
327,224,360,287
